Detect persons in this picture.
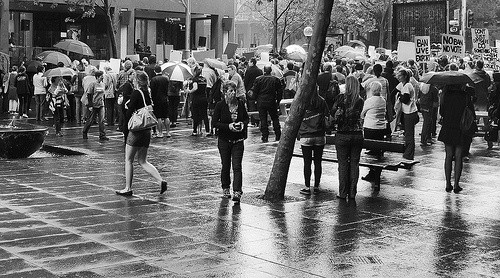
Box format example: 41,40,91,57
335,76,365,200
0,55,500,159
436,84,476,192
9,31,16,45
135,39,145,52
115,71,167,196
147,46,152,53
296,84,329,195
212,82,250,201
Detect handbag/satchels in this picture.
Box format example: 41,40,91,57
127,105,158,131
80,92,88,105
330,107,345,124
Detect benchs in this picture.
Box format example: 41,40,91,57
290,133,420,197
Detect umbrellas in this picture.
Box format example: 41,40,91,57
36,38,367,82
424,72,473,87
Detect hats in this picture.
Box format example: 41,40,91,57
135,70,149,86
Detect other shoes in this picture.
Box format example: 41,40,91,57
362,174,380,183
56,132,62,137
157,133,164,138
160,181,167,195
192,132,198,137
348,193,356,200
314,188,321,193
276,134,281,141
166,132,171,136
446,185,454,193
205,132,211,137
116,189,133,196
337,193,346,200
233,191,242,201
454,186,463,194
82,131,88,139
261,136,269,143
222,188,230,198
100,133,109,141
300,187,311,195
